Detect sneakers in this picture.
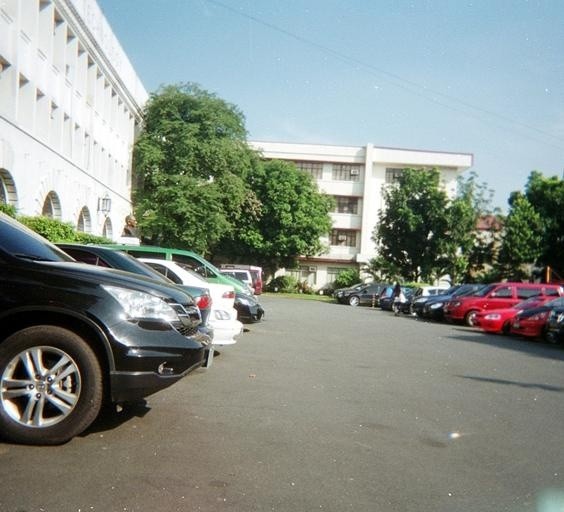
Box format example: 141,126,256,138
394,309,400,316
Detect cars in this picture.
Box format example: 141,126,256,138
332,283,564,344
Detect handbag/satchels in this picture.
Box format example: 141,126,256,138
399,289,406,304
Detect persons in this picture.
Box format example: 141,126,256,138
389,269,409,315
121,215,138,237
